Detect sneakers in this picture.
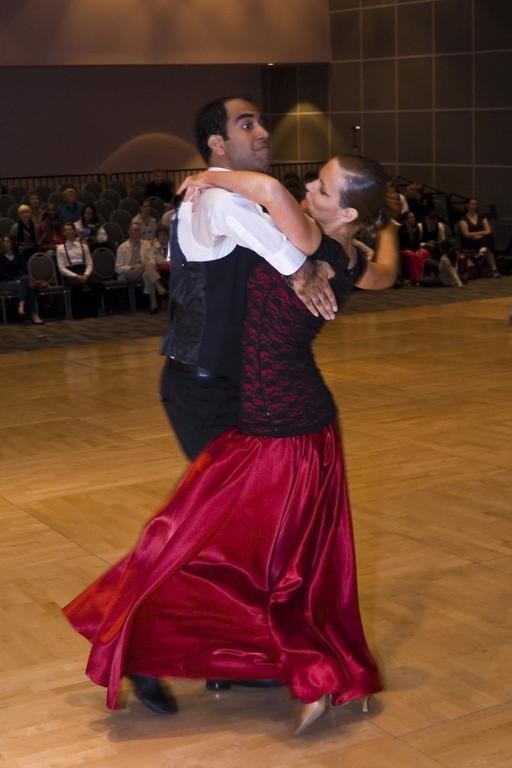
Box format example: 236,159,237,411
475,251,501,278
17,307,45,324
149,289,167,315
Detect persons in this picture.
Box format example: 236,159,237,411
61,154,408,737
125,97,409,712
401,198,500,287
1,171,177,324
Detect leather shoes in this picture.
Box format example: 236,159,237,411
205,679,285,691
125,670,176,716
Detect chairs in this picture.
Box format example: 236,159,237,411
1,179,177,325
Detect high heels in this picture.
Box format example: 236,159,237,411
290,691,375,735
